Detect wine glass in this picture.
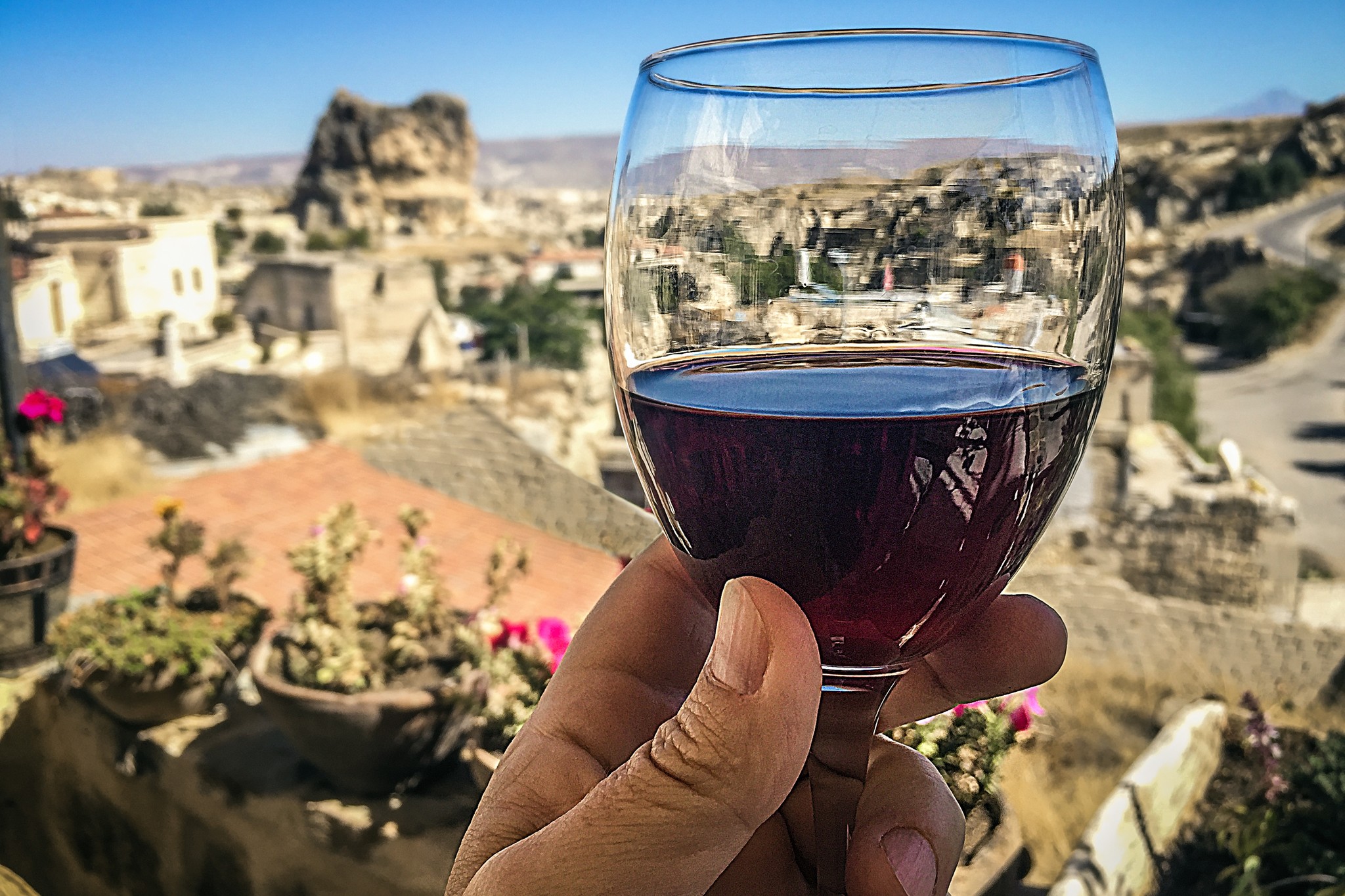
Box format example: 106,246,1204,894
602,27,1126,896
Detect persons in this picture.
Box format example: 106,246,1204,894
443,534,1069,896
